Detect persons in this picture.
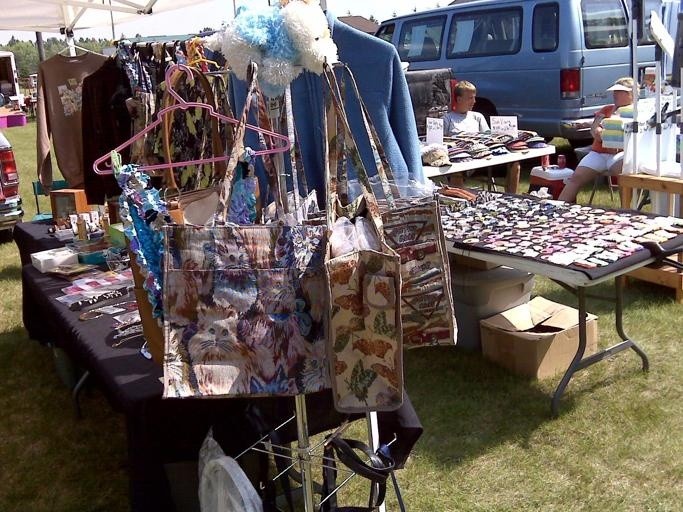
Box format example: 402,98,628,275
439,81,521,194
557,77,641,202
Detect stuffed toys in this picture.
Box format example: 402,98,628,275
208,1,339,98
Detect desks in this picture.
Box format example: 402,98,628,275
439,189,683,420
420,145,556,193
21,265,424,512
13,217,105,265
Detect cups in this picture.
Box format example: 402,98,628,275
100,217,108,228
76,221,88,239
167,201,183,225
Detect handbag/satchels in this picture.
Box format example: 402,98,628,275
261,84,320,226
322,63,404,413
340,60,458,350
122,66,226,367
163,62,324,400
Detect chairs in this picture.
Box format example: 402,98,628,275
32,180,70,223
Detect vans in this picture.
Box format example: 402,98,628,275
373,0,655,137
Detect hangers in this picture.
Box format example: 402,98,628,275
57,27,89,55
109,41,130,61
160,46,229,92
93,65,293,175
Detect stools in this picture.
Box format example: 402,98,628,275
528,164,577,205
587,173,618,204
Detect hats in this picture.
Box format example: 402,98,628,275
607,77,641,95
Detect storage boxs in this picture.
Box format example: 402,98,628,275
479,294,599,383
449,261,535,349
